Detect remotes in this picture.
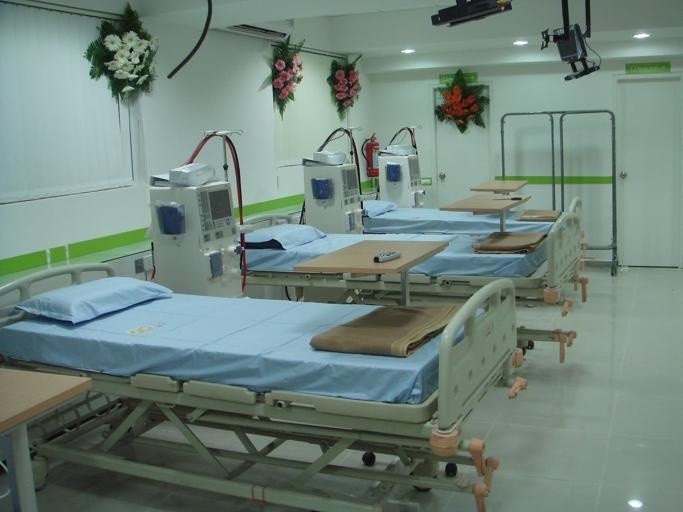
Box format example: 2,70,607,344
374,251,400,262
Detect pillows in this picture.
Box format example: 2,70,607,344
15,277,174,325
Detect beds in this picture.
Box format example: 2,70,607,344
237,213,581,363
0,262,576,512
354,198,588,307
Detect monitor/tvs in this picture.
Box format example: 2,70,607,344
208,189,232,221
553,24,587,61
412,159,418,174
346,168,358,190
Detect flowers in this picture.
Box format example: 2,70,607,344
272,35,305,120
327,54,362,121
434,68,489,134
82,3,157,105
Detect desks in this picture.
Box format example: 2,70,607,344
0,369,94,512
470,180,528,195
